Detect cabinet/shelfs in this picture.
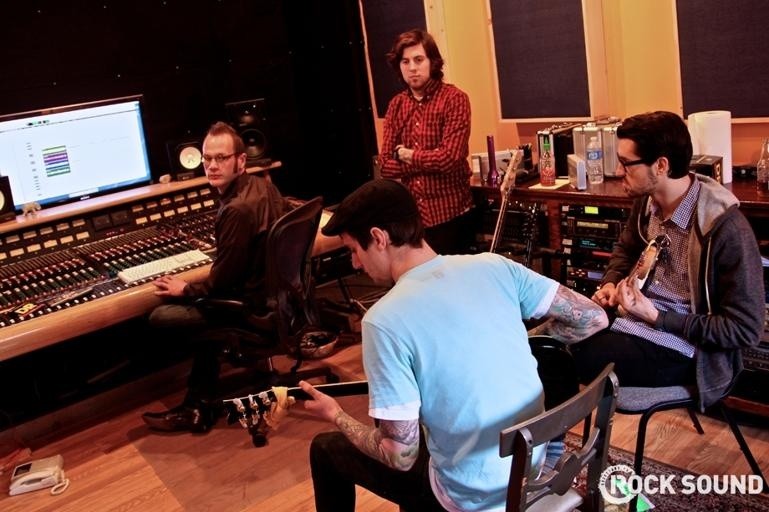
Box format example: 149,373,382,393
468,173,769,416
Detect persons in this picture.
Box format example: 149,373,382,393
533,111,765,475
141,121,293,434
378,30,476,252
299,178,617,511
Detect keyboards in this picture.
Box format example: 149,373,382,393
117,249,213,286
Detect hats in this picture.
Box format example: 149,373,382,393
320,178,418,237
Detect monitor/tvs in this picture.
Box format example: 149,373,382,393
0,95,152,212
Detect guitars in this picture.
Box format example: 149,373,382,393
223,334,580,447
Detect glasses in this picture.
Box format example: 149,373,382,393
616,151,652,167
202,151,239,164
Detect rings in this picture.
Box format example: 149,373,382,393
598,294,605,301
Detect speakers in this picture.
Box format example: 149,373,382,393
225,98,269,160
0,177,16,224
168,130,204,180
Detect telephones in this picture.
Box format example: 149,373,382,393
9,455,64,496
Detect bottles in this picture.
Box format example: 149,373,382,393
756,136,769,198
585,136,603,185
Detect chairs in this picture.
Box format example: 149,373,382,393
196,196,340,425
401,361,620,512
615,385,769,512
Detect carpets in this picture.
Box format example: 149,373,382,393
563,431,769,512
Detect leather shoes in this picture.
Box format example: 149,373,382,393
141,407,209,439
